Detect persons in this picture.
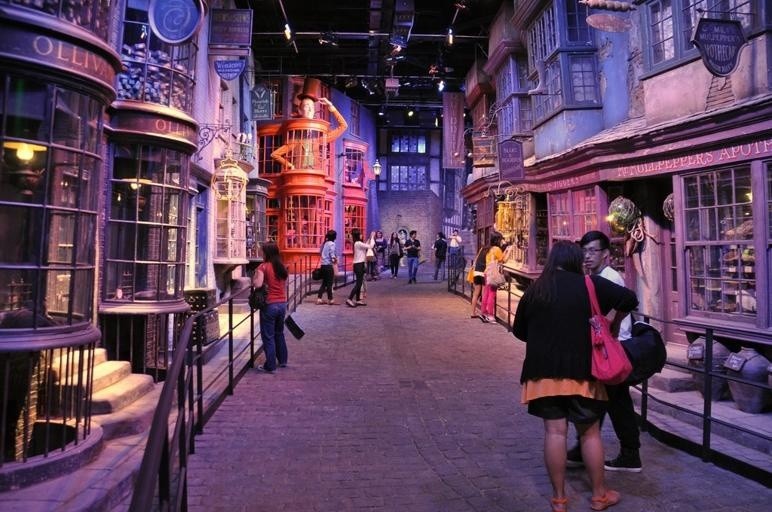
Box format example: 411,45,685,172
477,231,515,325
350,227,368,301
402,229,421,285
251,241,289,373
388,231,401,280
511,238,643,511
562,228,643,474
316,230,338,305
268,75,348,247
375,229,387,280
345,229,376,307
432,231,447,281
468,238,509,319
449,227,462,270
430,232,449,270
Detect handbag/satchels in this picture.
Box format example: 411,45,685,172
486,246,506,287
618,320,668,387
466,265,475,284
312,267,323,280
417,249,427,265
365,248,377,262
397,240,403,259
583,275,635,385
247,271,267,310
284,311,305,340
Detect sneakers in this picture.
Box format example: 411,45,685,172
566,443,584,467
257,364,277,374
479,313,497,323
279,364,286,368
408,279,412,284
389,274,397,280
602,447,643,473
412,277,416,284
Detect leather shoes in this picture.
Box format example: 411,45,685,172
355,302,367,306
346,299,357,308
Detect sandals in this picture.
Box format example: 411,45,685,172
550,495,569,512
316,299,327,305
590,490,622,509
329,299,341,305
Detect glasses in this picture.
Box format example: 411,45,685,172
582,247,604,255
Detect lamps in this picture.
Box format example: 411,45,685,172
194,123,249,201
369,159,382,182
2,130,47,161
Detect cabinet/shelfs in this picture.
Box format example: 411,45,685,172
692,217,756,316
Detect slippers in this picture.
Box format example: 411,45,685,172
471,313,480,318
